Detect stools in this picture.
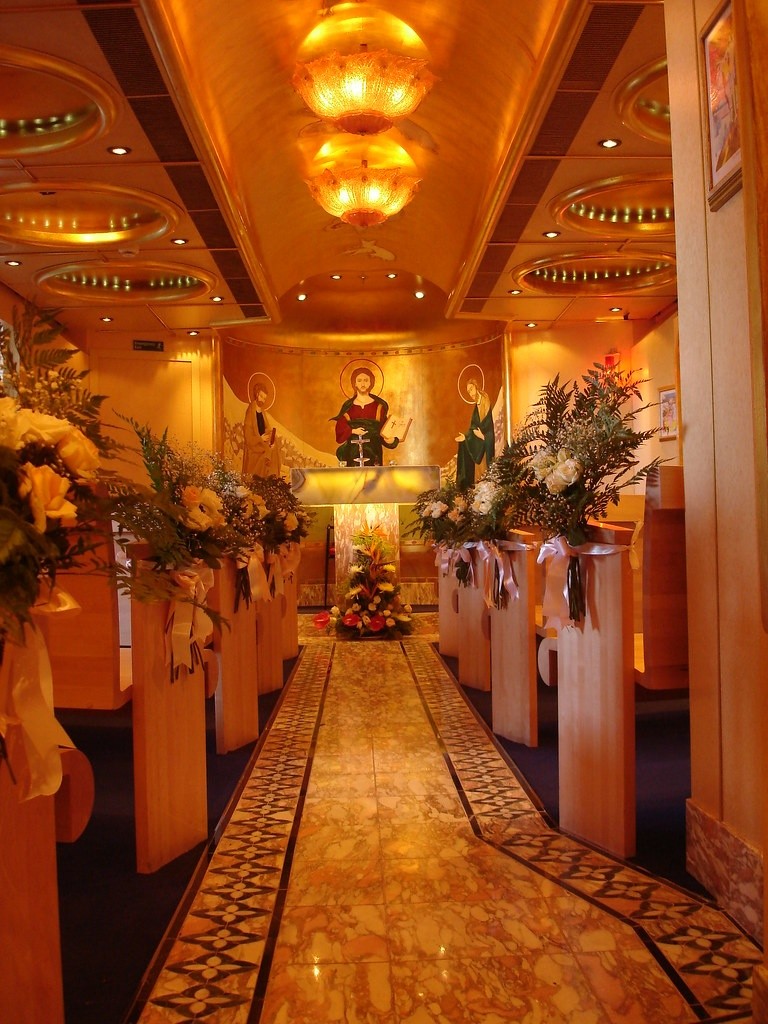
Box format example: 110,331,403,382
323,523,334,608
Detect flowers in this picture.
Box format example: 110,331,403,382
0,290,127,807
108,403,313,684
402,361,678,625
313,520,417,641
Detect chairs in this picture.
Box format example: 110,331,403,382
32,476,135,712
633,466,690,692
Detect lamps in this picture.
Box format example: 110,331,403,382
303,159,424,231
290,42,443,137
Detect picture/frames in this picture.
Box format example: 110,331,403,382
656,384,679,443
697,1,748,214
